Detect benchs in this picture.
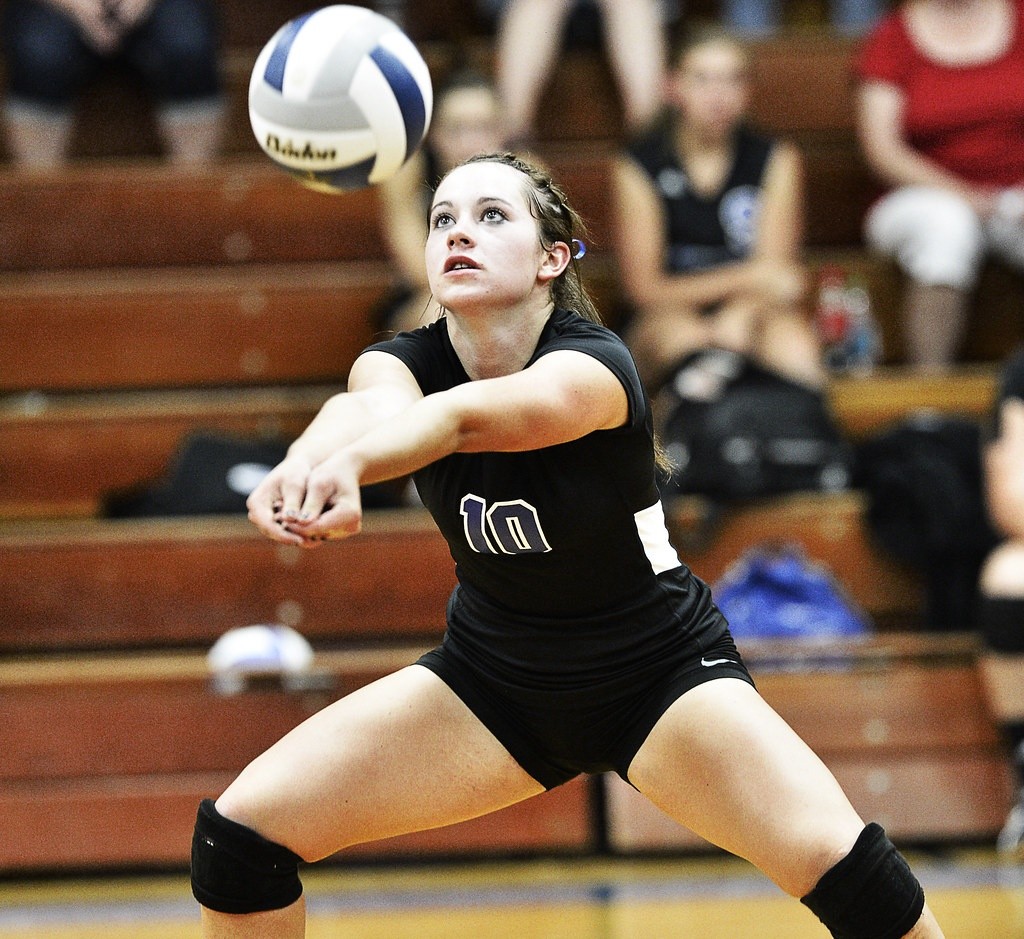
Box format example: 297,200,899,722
0,0,1022,875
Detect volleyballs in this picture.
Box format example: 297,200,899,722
246,2,436,196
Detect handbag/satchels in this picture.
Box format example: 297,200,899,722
669,363,837,496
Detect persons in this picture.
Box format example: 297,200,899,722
186,149,950,939
0,0,228,171
358,0,1023,859
